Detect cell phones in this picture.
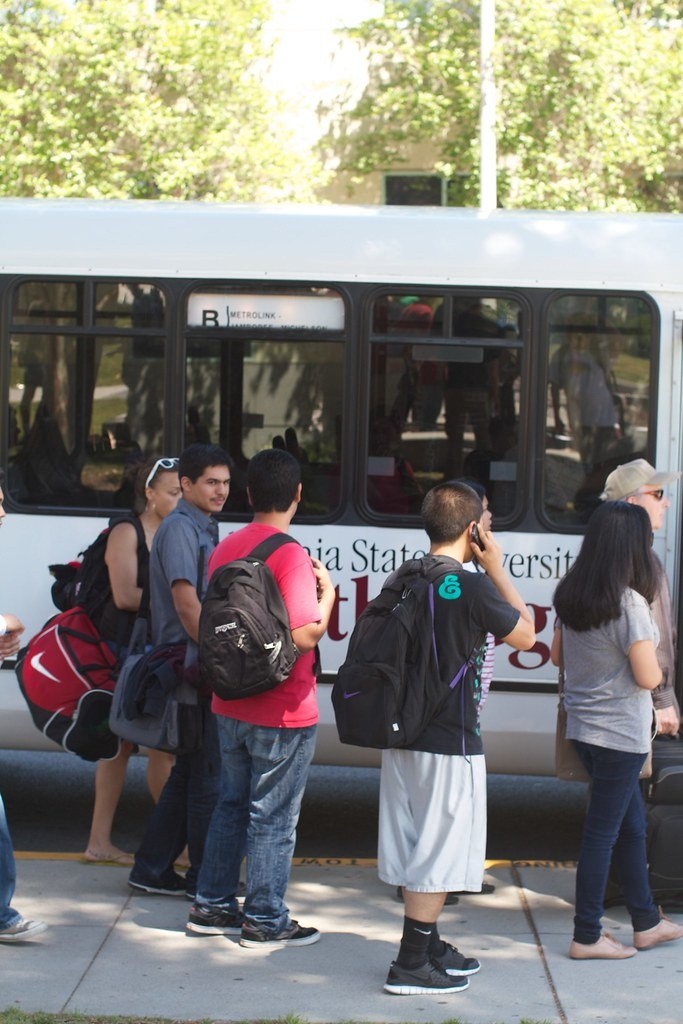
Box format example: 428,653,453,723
471,524,485,552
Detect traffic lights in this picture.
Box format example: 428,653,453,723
383,170,442,313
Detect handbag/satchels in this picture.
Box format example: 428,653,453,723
552,699,591,783
13,604,123,763
108,649,203,754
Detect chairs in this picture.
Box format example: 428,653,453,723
3,409,658,523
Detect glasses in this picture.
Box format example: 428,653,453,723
145,457,180,488
639,490,664,499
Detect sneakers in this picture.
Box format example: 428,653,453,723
128,864,188,895
383,956,471,995
185,900,245,935
186,881,247,903
432,938,481,975
240,918,320,947
0,919,48,942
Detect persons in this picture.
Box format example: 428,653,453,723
0,484,47,942
463,416,521,517
602,458,683,737
373,294,520,455
376,479,536,995
80,455,192,871
186,449,336,949
552,501,683,959
548,312,624,482
17,300,51,434
328,415,424,516
128,445,246,904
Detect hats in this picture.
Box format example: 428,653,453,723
604,457,682,500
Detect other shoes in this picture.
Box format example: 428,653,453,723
569,930,638,959
453,884,496,893
662,903,683,912
605,897,626,907
397,890,460,906
634,919,683,950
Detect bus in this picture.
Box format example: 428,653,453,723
0,194,683,777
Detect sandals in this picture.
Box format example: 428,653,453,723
83,851,136,868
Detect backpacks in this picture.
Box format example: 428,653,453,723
330,563,485,749
200,531,304,700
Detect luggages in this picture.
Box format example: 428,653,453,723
642,724,683,900
